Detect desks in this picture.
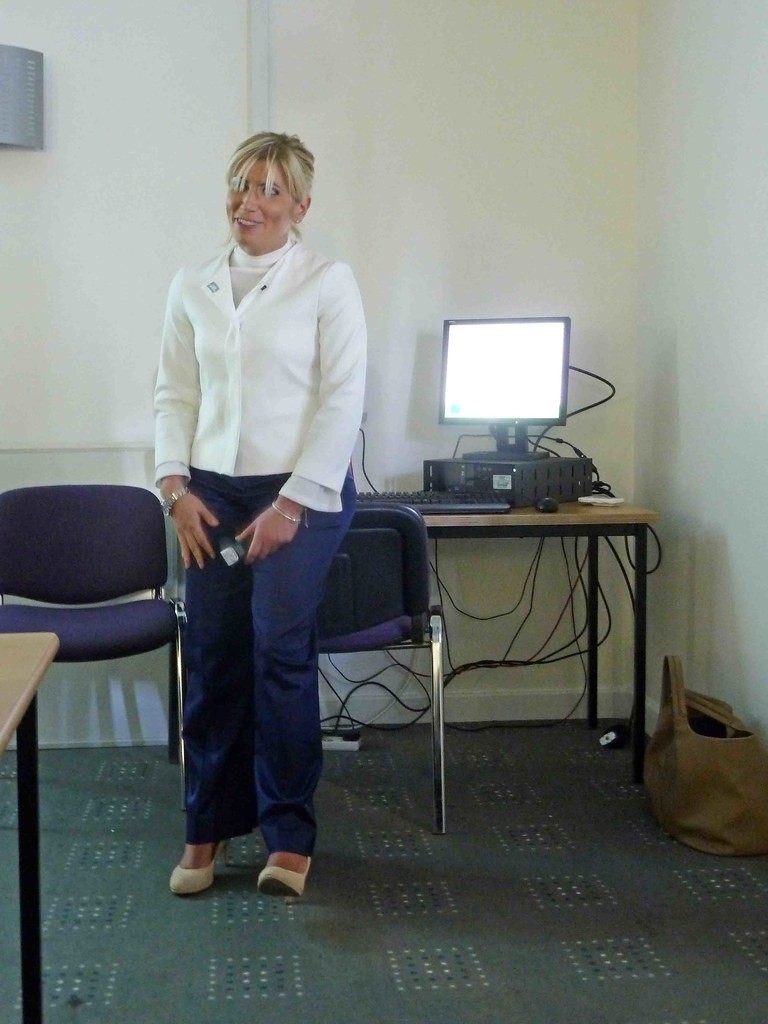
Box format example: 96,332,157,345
416,508,657,835
0,631,60,1024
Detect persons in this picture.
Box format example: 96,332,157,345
154,132,367,899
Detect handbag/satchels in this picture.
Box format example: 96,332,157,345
645,657,768,858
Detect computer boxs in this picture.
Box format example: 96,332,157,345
422,456,592,508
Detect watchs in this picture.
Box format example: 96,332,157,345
160,486,190,517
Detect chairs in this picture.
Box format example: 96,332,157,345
315,500,448,838
0,484,186,812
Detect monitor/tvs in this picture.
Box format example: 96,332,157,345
436,315,572,459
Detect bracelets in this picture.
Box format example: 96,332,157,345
272,502,302,523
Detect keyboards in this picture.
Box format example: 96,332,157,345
352,487,511,513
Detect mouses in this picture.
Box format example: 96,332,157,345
533,495,559,513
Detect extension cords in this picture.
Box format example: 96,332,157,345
321,735,361,751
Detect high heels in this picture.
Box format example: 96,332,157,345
258,853,310,896
169,838,231,894
